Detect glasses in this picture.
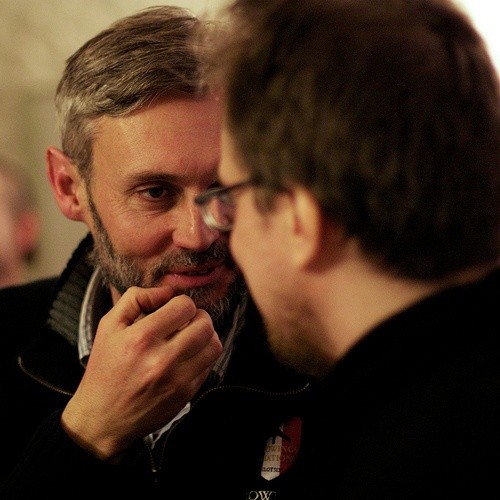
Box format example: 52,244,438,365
195,174,293,233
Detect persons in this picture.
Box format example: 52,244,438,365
0,150,45,290
195,1,500,500
1,4,315,500
